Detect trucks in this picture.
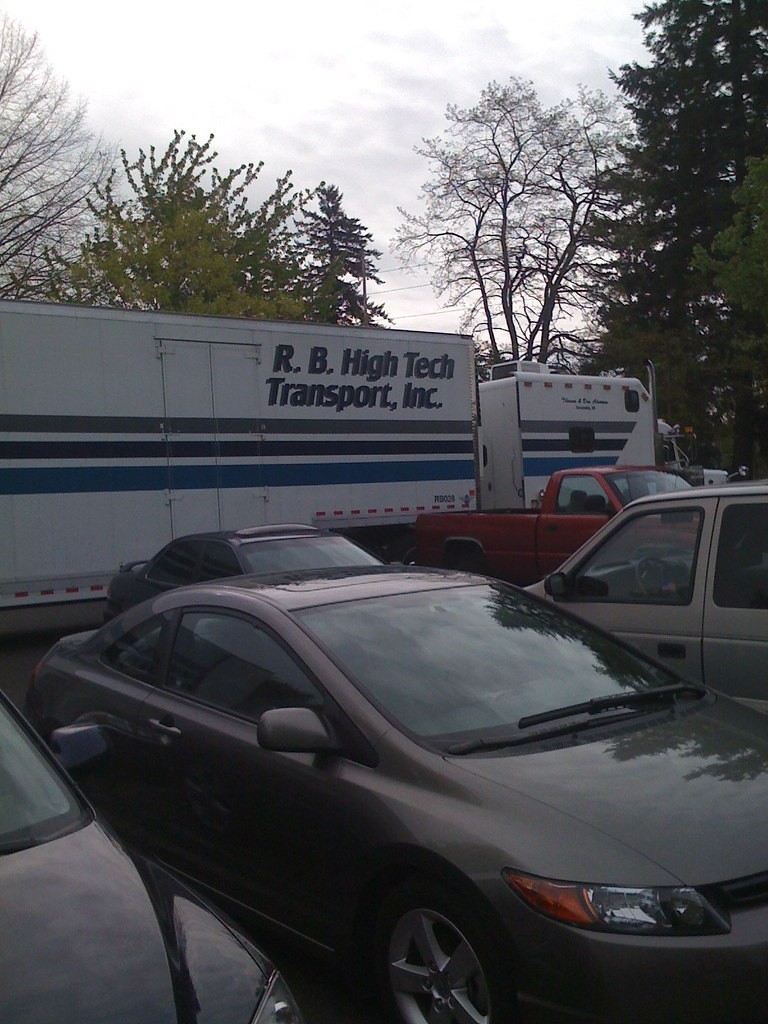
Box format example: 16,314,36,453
0,299,702,645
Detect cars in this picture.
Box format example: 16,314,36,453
0,689,305,1023
105,522,388,619
10,562,768,1024
512,477,768,708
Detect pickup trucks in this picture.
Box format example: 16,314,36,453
413,465,737,584
